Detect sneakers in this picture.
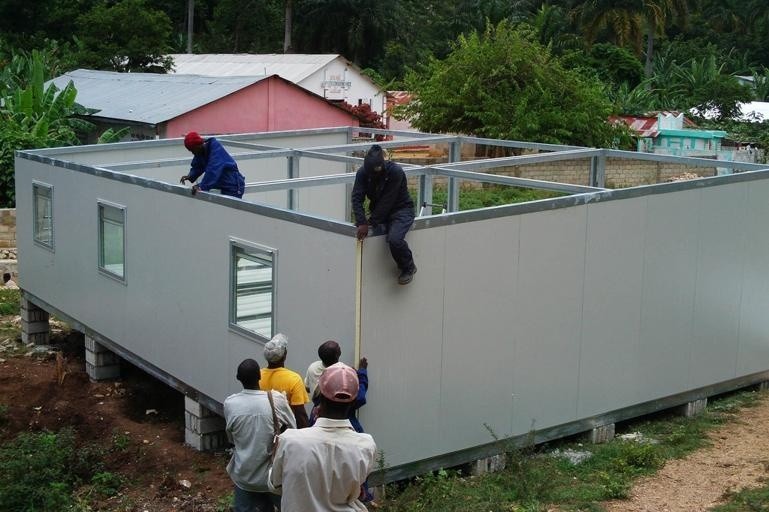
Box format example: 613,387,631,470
399,264,417,284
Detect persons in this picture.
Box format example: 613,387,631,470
302,340,347,403
259,332,310,428
268,360,376,511
307,356,377,505
179,130,245,200
353,144,419,284
221,359,297,511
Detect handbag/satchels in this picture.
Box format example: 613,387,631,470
267,390,280,463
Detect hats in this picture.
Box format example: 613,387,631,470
184,131,204,150
319,362,359,403
264,333,288,362
364,156,388,179
